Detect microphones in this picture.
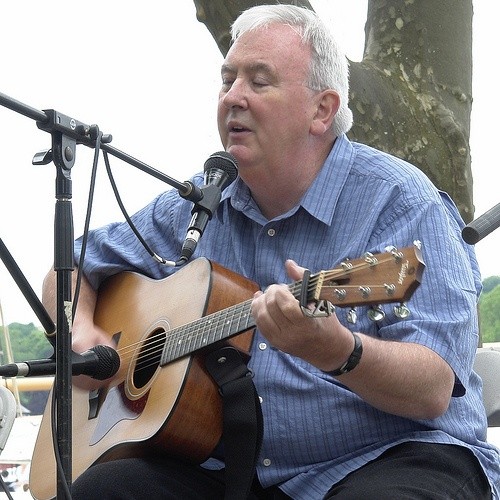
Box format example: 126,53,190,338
180,151,239,261
1,345,120,381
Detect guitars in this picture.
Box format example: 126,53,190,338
28,239,424,499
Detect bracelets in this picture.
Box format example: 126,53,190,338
318,328,363,378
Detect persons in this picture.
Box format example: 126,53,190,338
41,5,500,499
